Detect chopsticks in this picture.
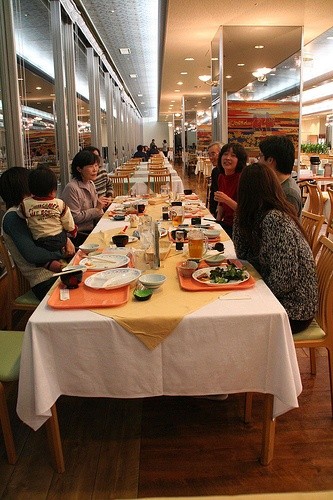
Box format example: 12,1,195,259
199,251,224,262
52,267,84,276
80,255,116,263
101,197,117,203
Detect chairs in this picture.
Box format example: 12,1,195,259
243,180,333,427
196,157,209,183
187,154,197,177
0,231,42,466
107,156,145,195
147,152,173,195
203,160,212,188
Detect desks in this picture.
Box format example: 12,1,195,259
195,160,204,175
111,169,178,175
112,174,185,194
304,191,331,224
148,157,168,161
118,164,173,168
123,159,170,164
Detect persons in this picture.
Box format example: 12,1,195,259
61,151,109,249
0,146,6,163
83,146,113,229
121,145,125,153
192,143,196,150
30,147,55,161
207,141,249,239
134,138,157,162
0,166,78,304
256,135,302,226
193,163,318,400
162,140,168,157
79,143,82,151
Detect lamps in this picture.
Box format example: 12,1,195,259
198,74,218,87
251,64,278,83
174,111,183,120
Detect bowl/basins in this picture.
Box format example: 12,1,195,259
139,273,167,289
78,243,100,255
112,236,128,247
177,260,198,277
62,265,87,275
61,271,83,289
202,254,225,267
204,229,220,239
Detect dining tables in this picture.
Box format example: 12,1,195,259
9,190,304,474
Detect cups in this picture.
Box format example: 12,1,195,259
160,185,169,198
162,205,185,226
122,201,154,247
187,230,209,259
176,231,185,251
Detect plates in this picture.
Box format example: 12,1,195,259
84,267,142,289
110,235,138,242
80,254,130,270
134,294,151,301
192,267,250,286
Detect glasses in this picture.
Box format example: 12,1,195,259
255,154,275,160
207,152,220,158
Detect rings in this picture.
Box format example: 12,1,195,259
217,194,218,196
